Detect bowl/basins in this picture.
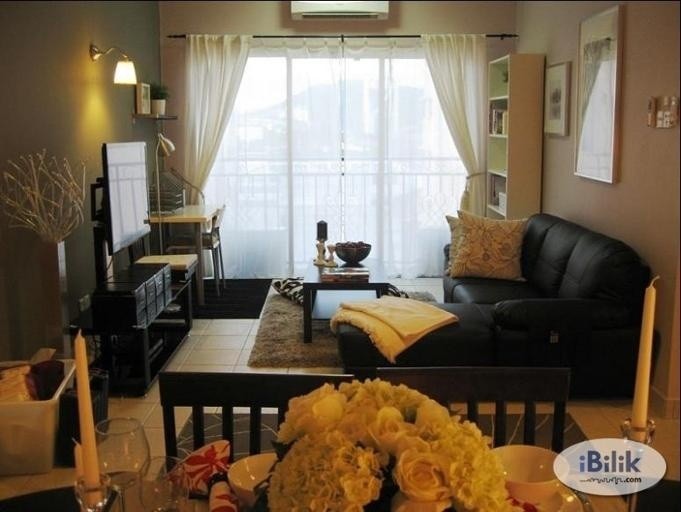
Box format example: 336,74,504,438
227,451,279,506
335,241,371,264
493,445,570,505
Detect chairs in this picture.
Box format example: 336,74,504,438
156,372,355,473
376,365,568,454
164,204,226,296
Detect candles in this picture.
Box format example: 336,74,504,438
630,275,661,442
74,328,100,487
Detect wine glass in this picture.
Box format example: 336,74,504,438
94,414,150,512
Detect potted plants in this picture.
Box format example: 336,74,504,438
145,79,170,116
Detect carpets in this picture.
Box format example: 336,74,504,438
156,410,589,478
247,290,438,366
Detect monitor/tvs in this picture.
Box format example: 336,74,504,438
101,142,151,257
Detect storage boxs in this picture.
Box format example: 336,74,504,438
90,262,172,315
0,359,76,477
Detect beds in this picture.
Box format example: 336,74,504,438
302,253,391,343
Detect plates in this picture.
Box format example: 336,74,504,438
531,483,592,512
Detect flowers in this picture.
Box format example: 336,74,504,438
262,376,524,512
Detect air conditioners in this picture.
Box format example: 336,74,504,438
290,0,390,23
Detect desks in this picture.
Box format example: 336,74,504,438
150,203,219,306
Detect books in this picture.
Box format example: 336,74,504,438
320,267,369,283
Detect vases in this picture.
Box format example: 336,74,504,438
40,243,69,356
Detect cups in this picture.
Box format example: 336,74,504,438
138,456,189,512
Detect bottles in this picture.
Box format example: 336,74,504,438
72,473,111,512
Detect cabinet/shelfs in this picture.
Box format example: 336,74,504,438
483,52,546,219
63,279,194,398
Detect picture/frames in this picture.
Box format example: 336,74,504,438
544,2,624,185
136,81,151,114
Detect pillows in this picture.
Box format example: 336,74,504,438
271,276,303,307
444,209,530,283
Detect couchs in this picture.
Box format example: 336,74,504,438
335,211,662,403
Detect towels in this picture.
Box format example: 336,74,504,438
329,294,459,365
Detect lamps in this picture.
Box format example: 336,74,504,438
154,132,176,254
90,43,138,86
313,220,328,266
